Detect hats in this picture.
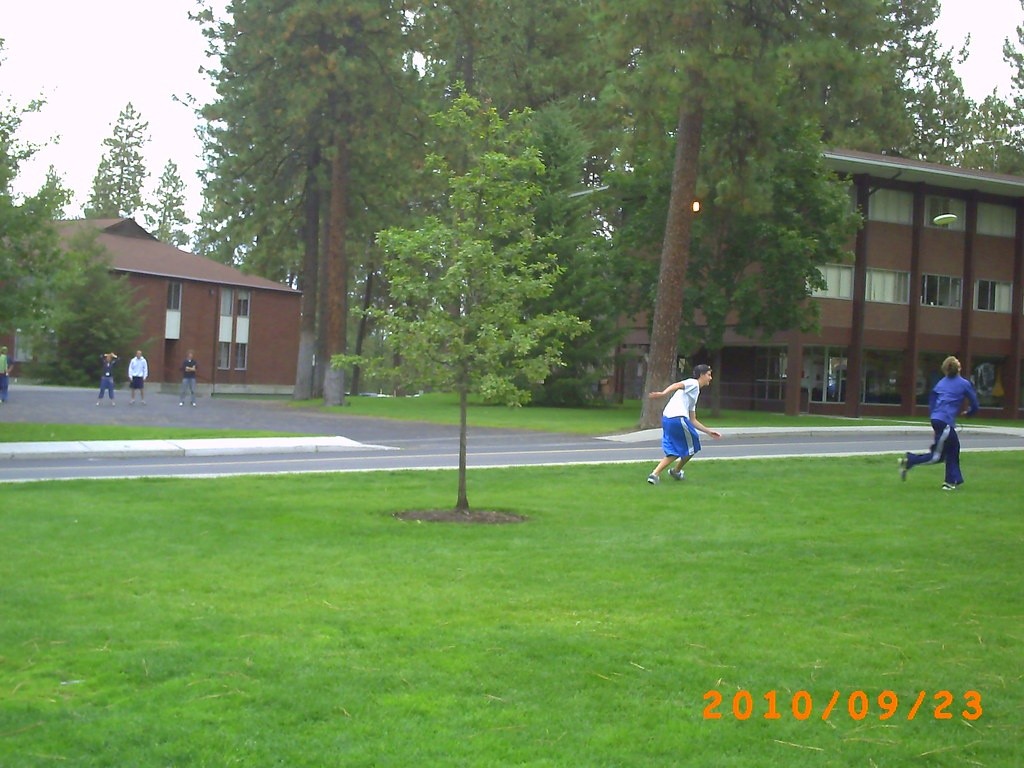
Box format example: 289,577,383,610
692,364,712,379
2,346,8,350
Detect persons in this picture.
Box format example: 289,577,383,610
178,349,198,407
896,356,979,491
95,352,120,406
128,350,148,406
645,364,721,485
0,346,14,404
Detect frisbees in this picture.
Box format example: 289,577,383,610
933,213,958,226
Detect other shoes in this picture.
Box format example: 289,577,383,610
193,402,196,406
0,399,2,403
141,400,145,405
96,401,100,406
112,401,116,406
129,400,134,404
179,402,183,406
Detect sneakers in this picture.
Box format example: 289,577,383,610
897,457,908,482
667,467,686,482
648,473,660,485
942,482,956,491
